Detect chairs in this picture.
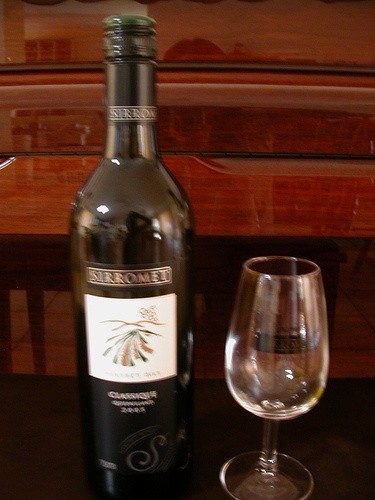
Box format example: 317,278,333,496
1,238,83,382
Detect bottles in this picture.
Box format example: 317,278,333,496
68,15,195,500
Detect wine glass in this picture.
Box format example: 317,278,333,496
219,255,329,499
